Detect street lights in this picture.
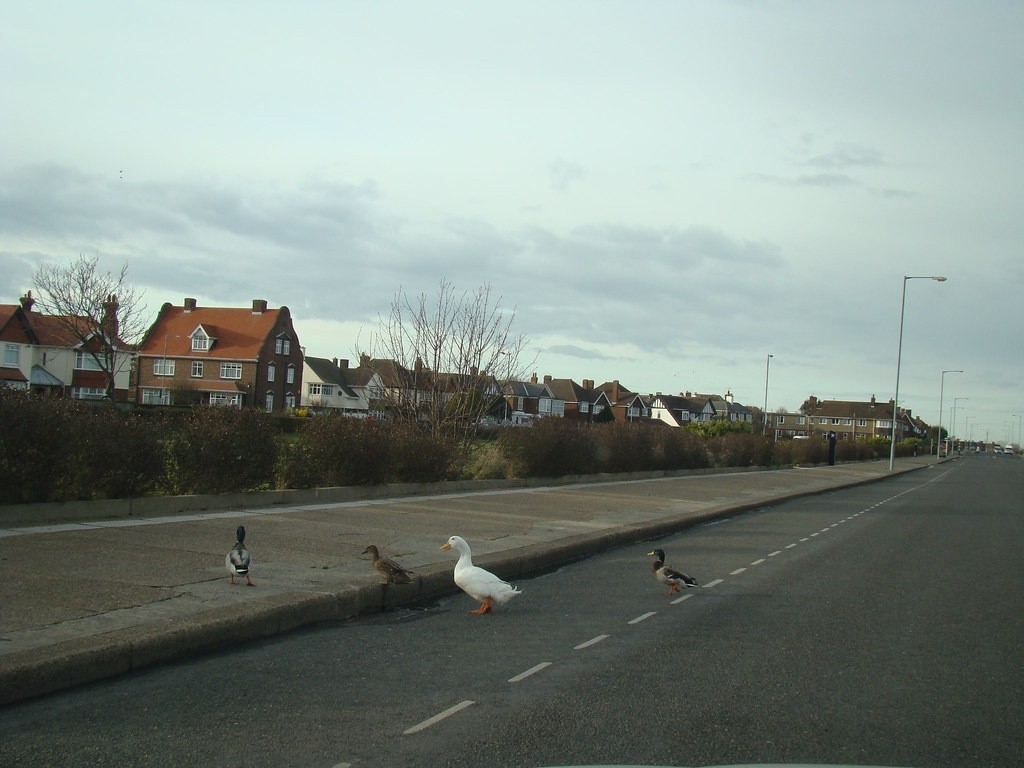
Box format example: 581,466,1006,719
500,351,510,420
1012,414,1021,446
936,369,963,463
888,275,948,472
952,397,969,456
763,354,773,435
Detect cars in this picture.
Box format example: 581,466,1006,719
993,446,1003,454
1005,445,1013,455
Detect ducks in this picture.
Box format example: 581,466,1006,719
225,526,258,587
361,545,416,586
439,535,524,615
647,549,698,597
